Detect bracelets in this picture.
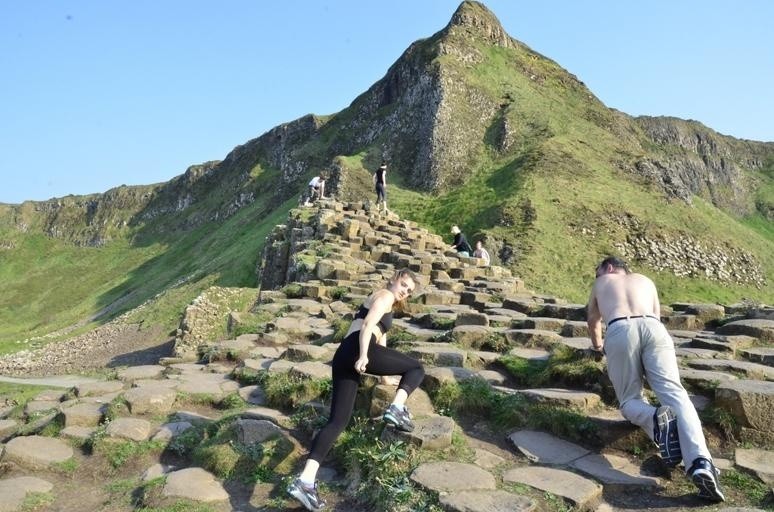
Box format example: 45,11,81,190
593,345,603,349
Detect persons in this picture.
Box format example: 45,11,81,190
586,257,726,504
373,163,389,211
305,175,327,205
449,226,472,257
287,267,426,512
473,240,490,264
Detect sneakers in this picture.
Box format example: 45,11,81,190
382,403,415,432
287,477,326,512
653,405,683,466
691,458,725,502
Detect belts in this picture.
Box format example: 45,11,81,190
608,315,659,326
